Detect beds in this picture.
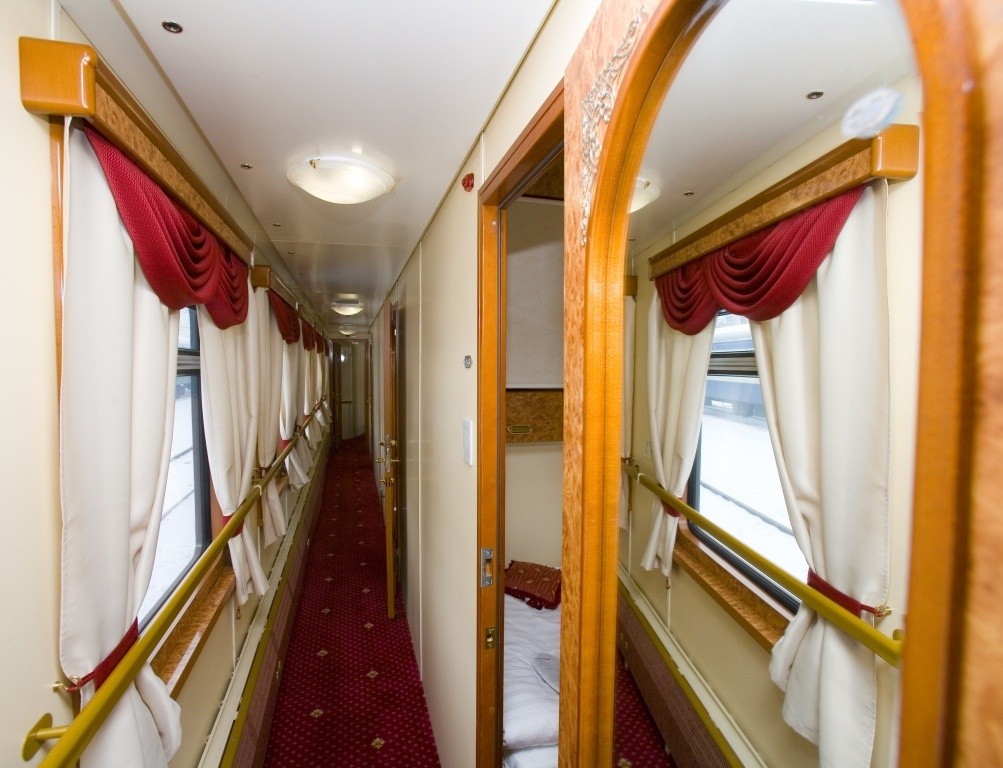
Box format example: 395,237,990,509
505,556,560,768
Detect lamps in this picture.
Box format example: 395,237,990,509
287,160,396,206
330,301,366,337
